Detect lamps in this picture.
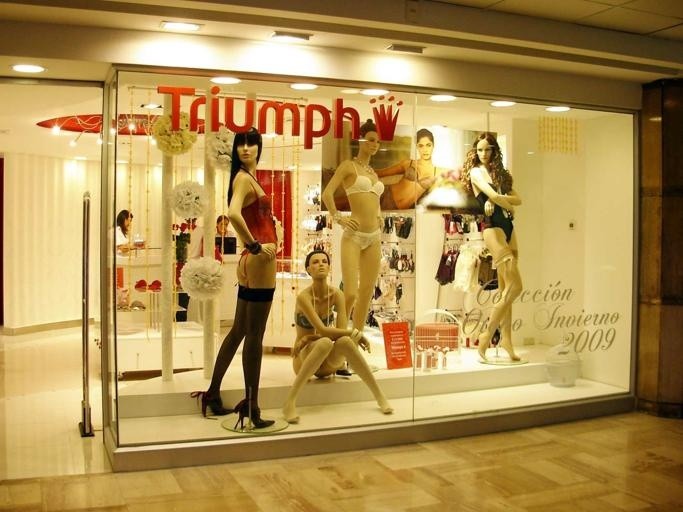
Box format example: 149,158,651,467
49,108,172,148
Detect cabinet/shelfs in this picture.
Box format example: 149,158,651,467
258,273,313,351
97,279,222,379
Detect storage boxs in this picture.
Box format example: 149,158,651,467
415,325,457,353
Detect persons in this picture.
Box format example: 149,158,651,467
107,209,134,249
321,128,449,209
281,248,393,424
459,131,522,362
200,126,277,429
215,215,235,238
320,120,384,335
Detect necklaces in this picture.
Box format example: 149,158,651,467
352,155,375,175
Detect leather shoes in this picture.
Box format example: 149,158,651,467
336,369,352,376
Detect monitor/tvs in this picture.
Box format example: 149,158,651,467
215,237,236,254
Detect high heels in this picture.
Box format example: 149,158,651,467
199,392,233,417
237,403,275,430
